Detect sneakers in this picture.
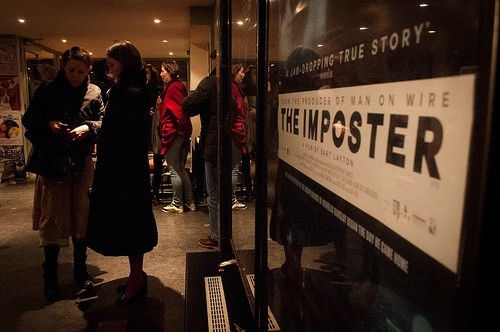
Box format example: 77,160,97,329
231,202,247,210
198,237,218,249
162,204,184,214
185,204,196,211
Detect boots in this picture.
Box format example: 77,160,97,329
42,261,59,299
72,254,94,288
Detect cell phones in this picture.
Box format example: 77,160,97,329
60,124,68,129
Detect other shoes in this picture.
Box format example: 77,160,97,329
154,195,159,205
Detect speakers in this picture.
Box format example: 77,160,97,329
189,5,213,25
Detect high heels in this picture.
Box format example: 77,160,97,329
115,272,148,304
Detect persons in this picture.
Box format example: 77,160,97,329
145,63,160,97
84,41,158,304
92,58,115,105
181,50,219,248
332,226,380,285
269,45,344,293
231,62,250,210
22,46,105,297
156,60,196,213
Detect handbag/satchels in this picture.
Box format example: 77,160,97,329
23,138,81,180
